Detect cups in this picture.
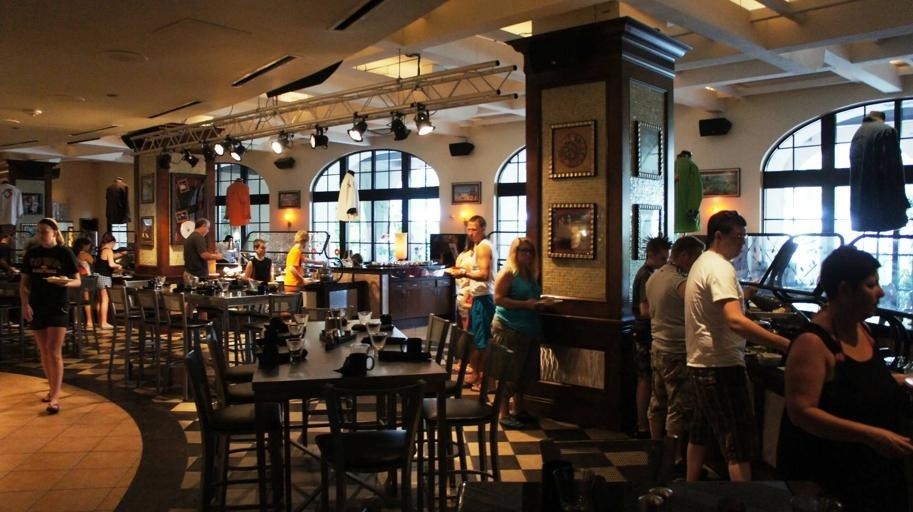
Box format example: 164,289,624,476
349,352,374,377
399,338,421,355
379,314,392,331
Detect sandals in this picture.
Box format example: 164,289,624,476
41,393,59,413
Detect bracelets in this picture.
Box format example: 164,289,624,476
458,268,466,278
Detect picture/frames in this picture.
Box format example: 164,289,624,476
278,190,300,209
699,168,741,198
175,210,190,223
176,178,190,194
22,192,43,214
140,216,154,247
634,119,666,181
548,120,595,178
141,173,154,203
547,203,595,260
633,204,664,260
452,181,481,204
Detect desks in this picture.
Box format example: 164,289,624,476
455,479,799,511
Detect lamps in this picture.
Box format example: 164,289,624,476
157,101,436,169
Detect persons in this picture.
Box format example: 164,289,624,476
285,229,327,314
444,234,474,375
350,253,367,269
183,217,224,339
336,169,363,223
242,239,277,334
772,244,912,512
96,231,128,330
674,149,704,234
71,236,96,329
223,178,252,228
1,180,23,224
681,210,791,483
643,233,710,480
18,216,83,414
1,221,22,330
489,238,555,431
442,214,497,393
849,110,908,232
632,229,671,451
106,175,131,225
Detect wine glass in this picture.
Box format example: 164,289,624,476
172,276,229,296
294,313,309,334
368,319,380,335
285,339,304,370
369,332,387,360
288,324,303,341
357,311,371,332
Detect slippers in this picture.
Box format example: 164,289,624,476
454,365,496,393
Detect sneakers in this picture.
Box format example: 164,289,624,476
500,416,525,429
101,323,114,330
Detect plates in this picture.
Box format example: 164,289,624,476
42,277,75,284
752,311,795,320
535,299,562,305
